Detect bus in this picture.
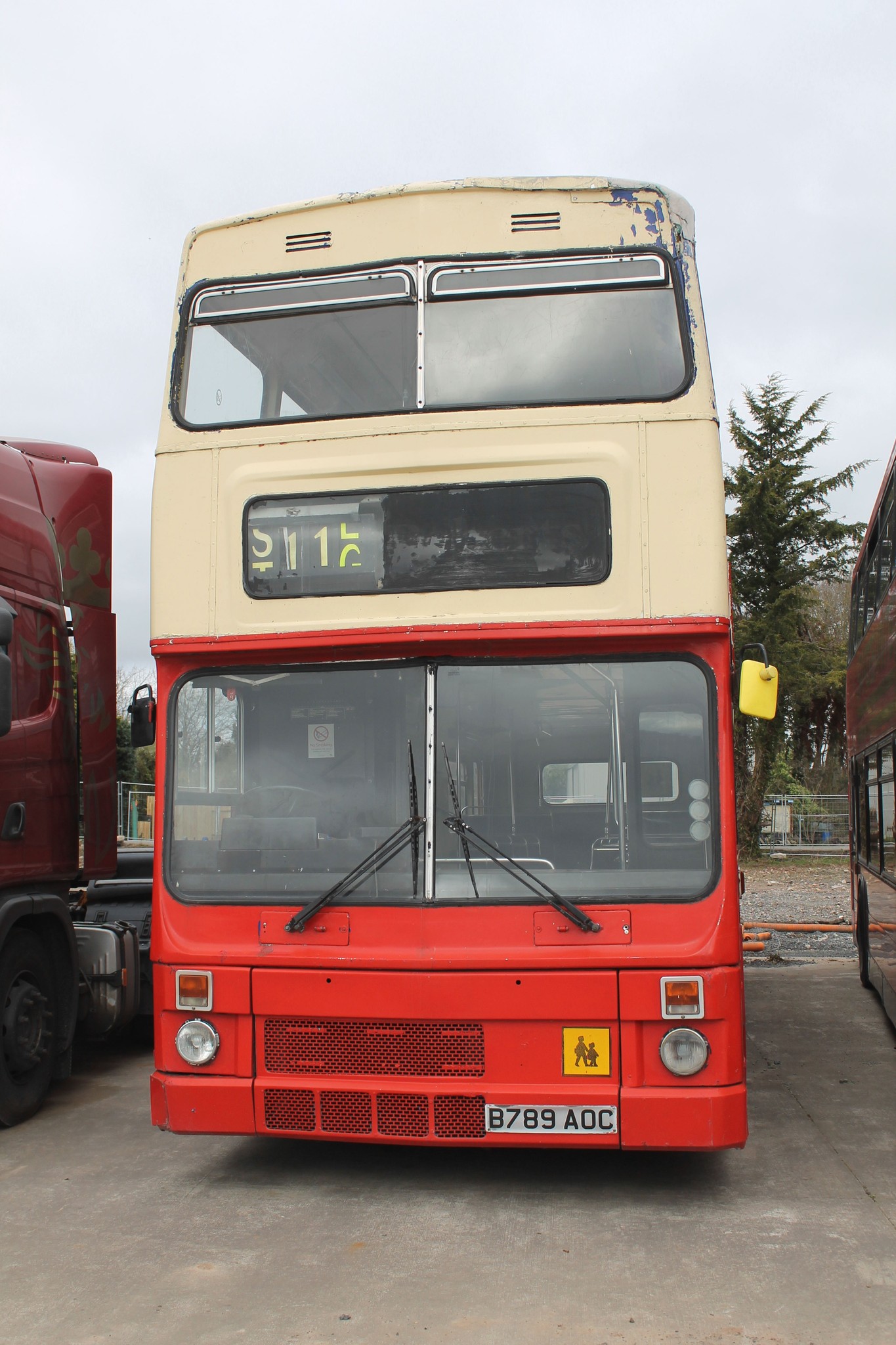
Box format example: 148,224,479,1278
845,439,896,1031
125,170,779,1152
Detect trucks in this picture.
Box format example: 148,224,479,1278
0,437,365,1135
